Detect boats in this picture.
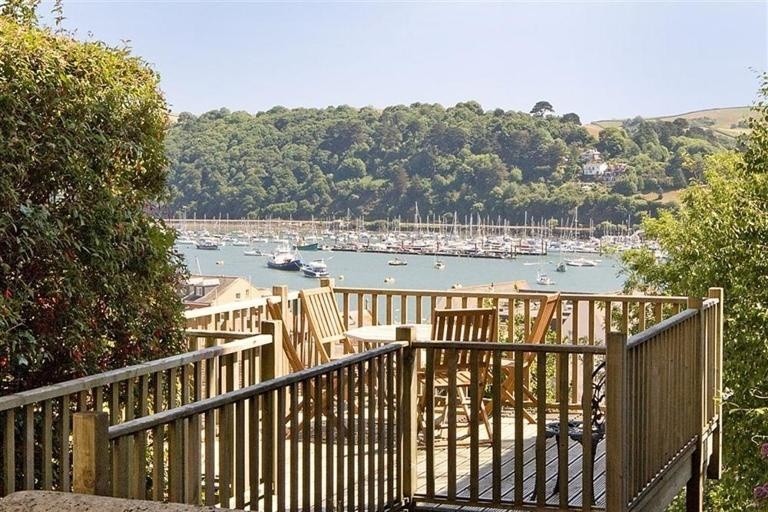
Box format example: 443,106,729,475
525,259,601,286
243,244,464,289
